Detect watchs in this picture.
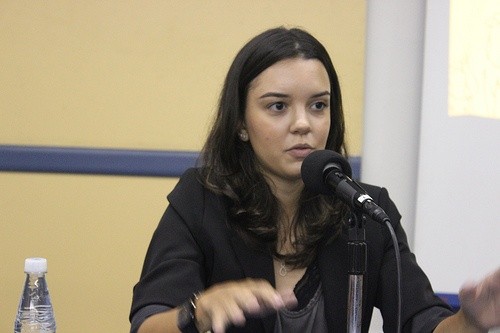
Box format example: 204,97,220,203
178,293,199,333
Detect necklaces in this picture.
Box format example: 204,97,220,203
275,252,301,276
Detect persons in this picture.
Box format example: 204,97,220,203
128,26,499,333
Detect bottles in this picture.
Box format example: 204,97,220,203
11,256,57,333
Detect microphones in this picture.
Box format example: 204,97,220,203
300,149,391,225
